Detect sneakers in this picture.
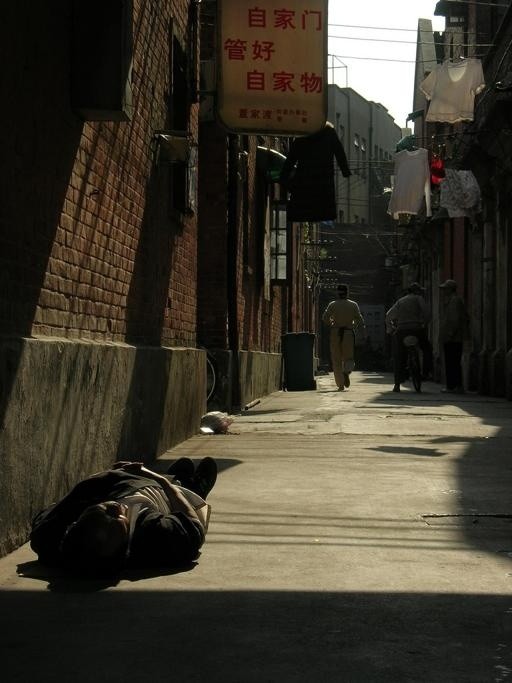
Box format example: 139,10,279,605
344,374,350,387
441,387,458,394
193,457,217,498
393,384,400,391
423,374,432,383
167,456,196,487
337,386,344,392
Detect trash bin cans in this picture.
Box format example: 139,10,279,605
281,332,316,391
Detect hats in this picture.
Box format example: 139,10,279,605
410,283,427,291
438,280,458,289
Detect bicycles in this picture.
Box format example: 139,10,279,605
403,335,425,391
195,342,218,404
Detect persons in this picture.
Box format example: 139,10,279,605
437,277,466,394
28,454,220,594
383,281,434,393
321,283,369,392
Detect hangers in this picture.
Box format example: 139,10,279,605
446,42,469,68
